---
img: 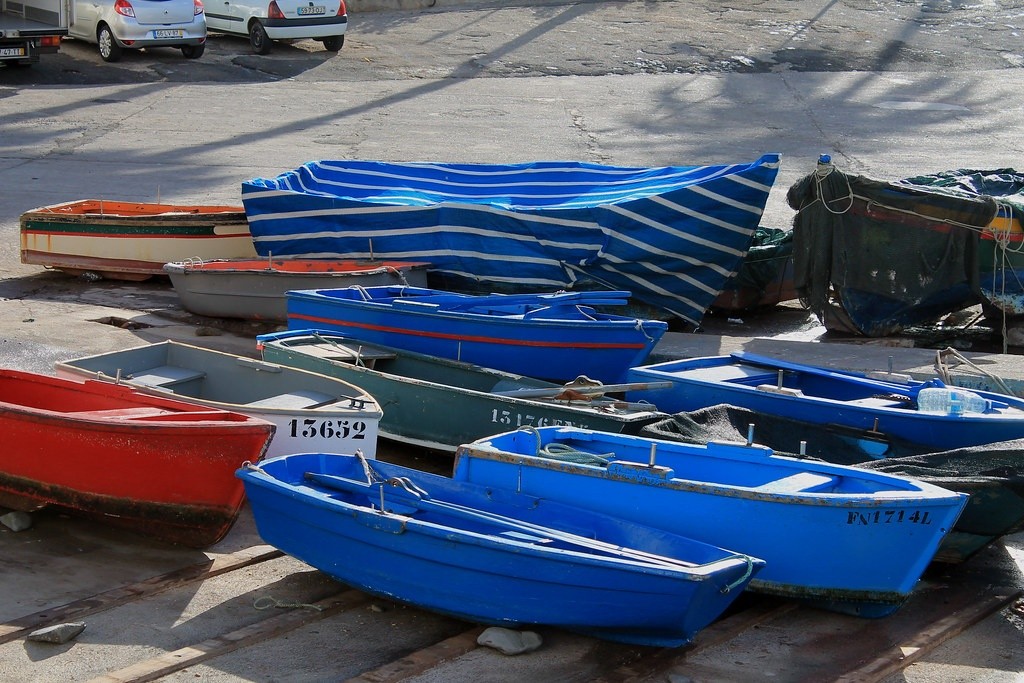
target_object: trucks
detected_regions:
[0,0,68,70]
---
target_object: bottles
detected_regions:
[917,388,991,415]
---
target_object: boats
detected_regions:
[234,449,768,653]
[241,151,782,326]
[0,369,278,550]
[623,351,1024,459]
[19,197,260,286]
[640,401,1024,567]
[786,153,1023,340]
[256,328,670,454]
[52,339,384,460]
[285,284,669,400]
[163,257,431,325]
[451,423,971,619]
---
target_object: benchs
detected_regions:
[123,365,205,398]
[847,397,907,408]
[755,472,842,493]
[676,364,804,394]
[291,342,397,370]
[246,390,336,409]
[69,407,180,420]
[496,530,552,546]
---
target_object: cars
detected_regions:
[201,0,348,56]
[63,0,208,62]
